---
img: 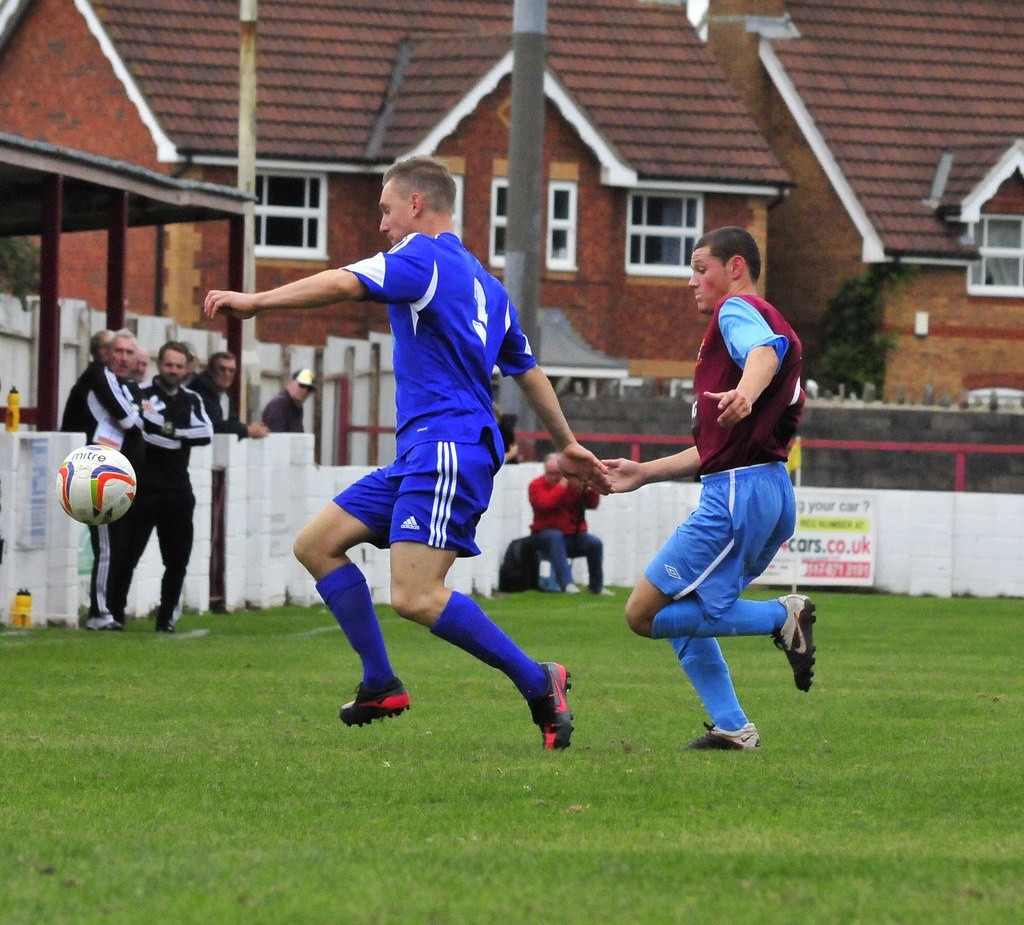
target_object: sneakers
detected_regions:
[769,594,816,692]
[339,677,410,727]
[526,662,574,749]
[682,722,759,750]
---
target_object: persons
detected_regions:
[602,227,817,750]
[201,156,612,750]
[57,328,316,633]
[528,453,616,595]
[492,401,520,463]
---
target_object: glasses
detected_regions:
[298,383,315,393]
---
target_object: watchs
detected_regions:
[163,421,174,435]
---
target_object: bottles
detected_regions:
[13,588,32,629]
[5,385,20,432]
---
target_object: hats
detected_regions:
[293,367,316,387]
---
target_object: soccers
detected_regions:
[55,444,138,526]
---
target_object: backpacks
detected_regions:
[500,538,538,591]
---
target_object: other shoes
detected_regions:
[87,621,124,631]
[566,582,581,594]
[587,586,614,594]
[156,624,176,632]
